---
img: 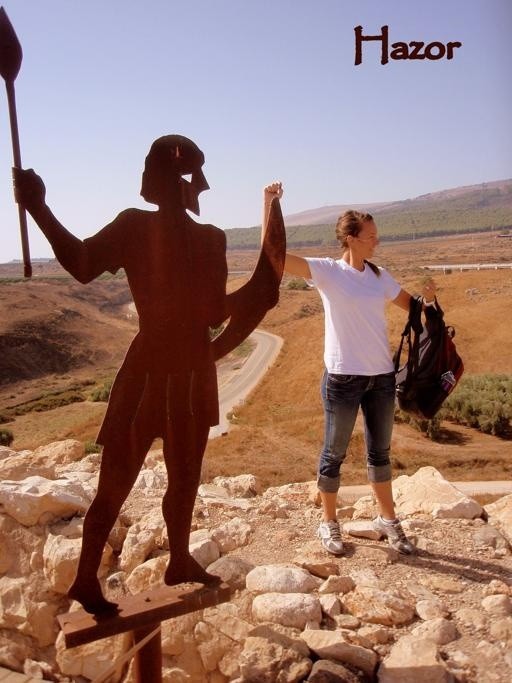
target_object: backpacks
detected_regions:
[391,294,466,421]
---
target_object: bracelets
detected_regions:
[422,297,435,306]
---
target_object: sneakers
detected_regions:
[315,520,347,554]
[371,513,415,555]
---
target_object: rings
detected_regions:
[426,285,430,290]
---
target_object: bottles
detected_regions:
[440,370,457,395]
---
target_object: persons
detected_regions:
[11,131,283,620]
[260,177,439,556]
